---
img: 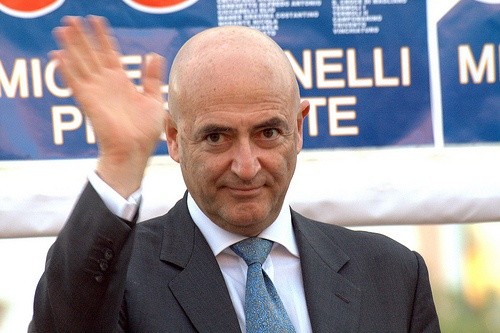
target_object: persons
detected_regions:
[27,11,442,333]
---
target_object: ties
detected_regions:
[230,237,296,333]
